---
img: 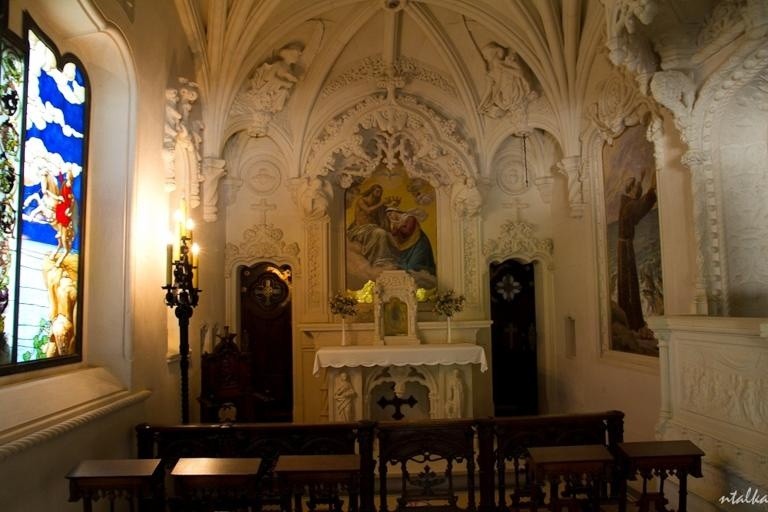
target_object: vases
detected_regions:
[342,318,345,346]
[447,316,451,343]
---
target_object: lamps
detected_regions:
[162,211,203,425]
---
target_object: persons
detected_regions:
[354,184,435,276]
[333,372,356,423]
[617,169,656,330]
[48,167,79,239]
[477,40,521,115]
[264,46,303,88]
[446,368,466,419]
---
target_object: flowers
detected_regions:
[428,290,466,317]
[328,290,360,318]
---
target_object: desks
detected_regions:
[313,344,488,422]
[616,440,705,512]
[65,454,361,512]
[526,445,614,512]
